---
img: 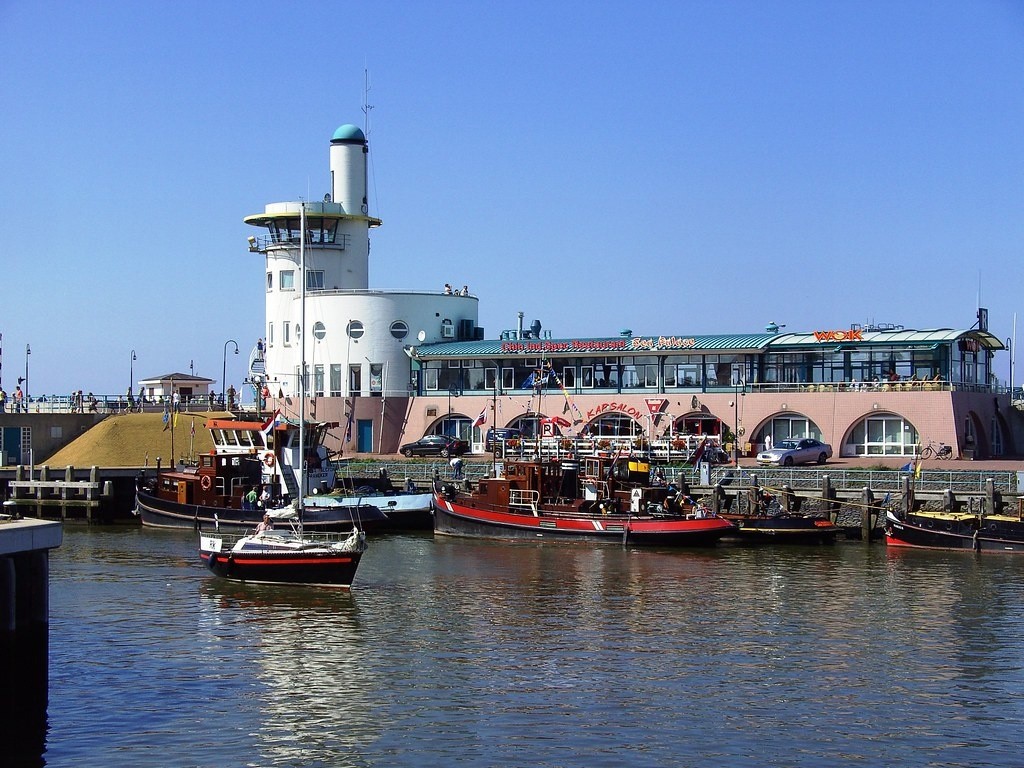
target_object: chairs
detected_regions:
[800,374,940,392]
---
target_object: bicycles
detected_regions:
[921,436,953,460]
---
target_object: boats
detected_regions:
[182,411,437,526]
[134,449,390,537]
[886,504,1024,552]
[578,454,845,541]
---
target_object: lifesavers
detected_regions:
[587,478,596,486]
[470,490,481,499]
[264,452,275,466]
[201,475,212,489]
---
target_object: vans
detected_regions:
[486,426,533,458]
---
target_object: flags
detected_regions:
[472,405,487,428]
[882,490,890,508]
[900,461,912,472]
[521,370,549,389]
[915,461,922,479]
[685,440,706,465]
[261,409,281,436]
[163,409,178,431]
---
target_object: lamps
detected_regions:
[388,501,397,506]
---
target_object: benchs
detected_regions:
[107,403,137,413]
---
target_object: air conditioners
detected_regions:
[442,325,454,337]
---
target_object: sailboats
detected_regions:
[432,345,738,547]
[197,200,368,593]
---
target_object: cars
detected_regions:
[755,438,835,465]
[399,434,470,457]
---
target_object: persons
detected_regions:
[653,435,662,450]
[257,337,266,362]
[0,385,48,414]
[71,390,86,414]
[890,368,899,386]
[89,392,99,413]
[637,430,647,440]
[109,387,172,415]
[450,458,463,480]
[241,486,271,511]
[190,385,236,404]
[255,375,270,410]
[444,283,469,297]
[849,379,880,392]
[173,389,181,414]
[764,433,771,450]
[255,514,274,535]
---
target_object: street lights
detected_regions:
[448,380,460,461]
[734,377,746,467]
[26,343,32,413]
[222,340,240,409]
[130,350,135,398]
[190,359,194,375]
